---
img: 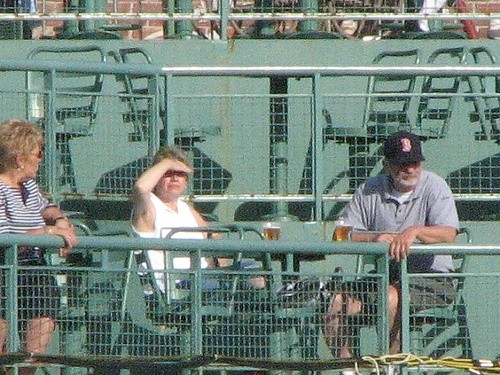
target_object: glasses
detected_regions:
[27,151,42,158]
[164,171,185,176]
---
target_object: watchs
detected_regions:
[53,216,70,226]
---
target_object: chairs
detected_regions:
[108,46,219,152]
[65,231,134,375]
[24,45,106,195]
[207,225,319,374]
[322,227,472,361]
[368,46,466,192]
[461,46,500,145]
[162,226,244,373]
[321,50,420,195]
[111,230,180,374]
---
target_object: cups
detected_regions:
[334,221,354,242]
[263,223,281,241]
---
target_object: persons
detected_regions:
[126,143,322,312]
[0,117,77,375]
[315,129,461,375]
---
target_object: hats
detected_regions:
[384,132,425,166]
